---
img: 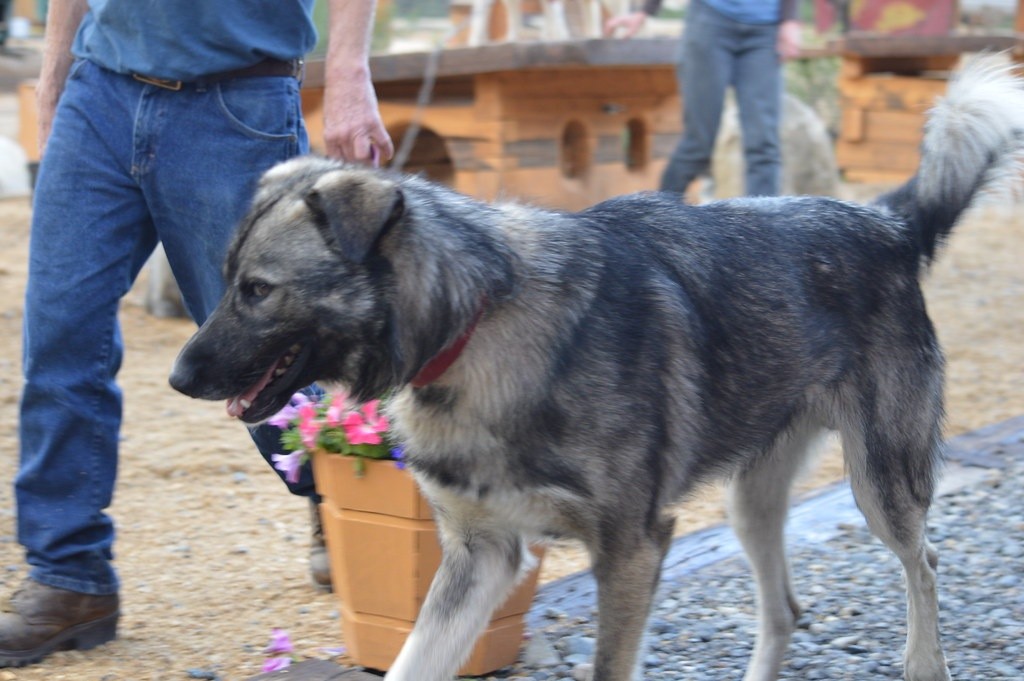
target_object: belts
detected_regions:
[128,62,294,77]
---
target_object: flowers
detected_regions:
[262,380,410,487]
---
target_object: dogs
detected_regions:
[165,47,1021,681]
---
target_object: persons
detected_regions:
[0,0,396,668]
[600,0,805,200]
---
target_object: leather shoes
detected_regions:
[0,577,119,668]
[306,495,335,591]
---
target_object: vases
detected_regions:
[308,445,557,679]
